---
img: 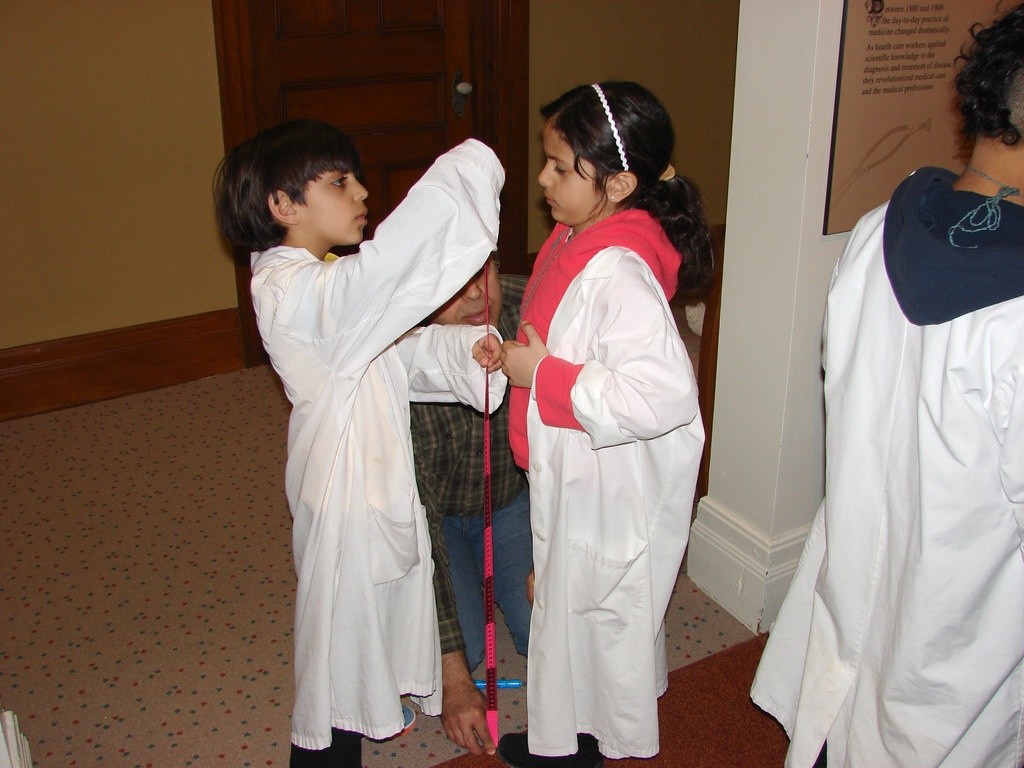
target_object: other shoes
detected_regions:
[498,729,604,768]
[371,703,417,739]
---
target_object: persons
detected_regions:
[748,1,1024,768]
[215,82,714,767]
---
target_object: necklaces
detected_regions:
[518,215,573,325]
[949,162,1024,248]
